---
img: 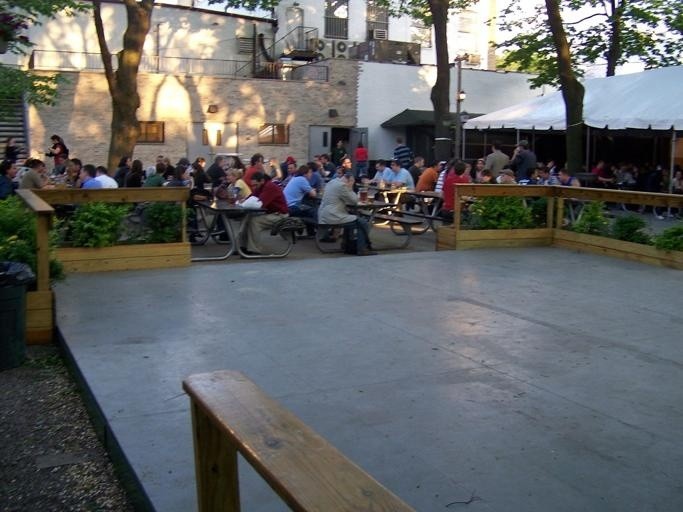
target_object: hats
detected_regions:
[176,158,189,166]
[287,157,295,163]
[515,140,528,147]
[499,169,513,177]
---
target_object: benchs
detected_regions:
[280,199,444,255]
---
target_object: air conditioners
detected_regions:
[309,38,353,62]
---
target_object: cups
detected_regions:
[321,183,326,195]
[358,187,369,204]
[228,189,237,204]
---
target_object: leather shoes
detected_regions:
[357,250,378,255]
[233,247,247,256]
[240,251,259,259]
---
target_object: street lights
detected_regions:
[454,53,469,163]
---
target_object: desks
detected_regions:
[197,201,265,262]
[355,183,444,199]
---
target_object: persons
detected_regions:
[352,142,367,174]
[408,156,583,225]
[592,161,681,219]
[392,137,415,167]
[43,134,68,166]
[5,136,21,163]
[330,138,347,163]
[483,140,509,177]
[508,139,535,177]
[1,154,118,216]
[114,155,414,253]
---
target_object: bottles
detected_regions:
[377,179,385,188]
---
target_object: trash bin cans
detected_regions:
[0,261,36,370]
